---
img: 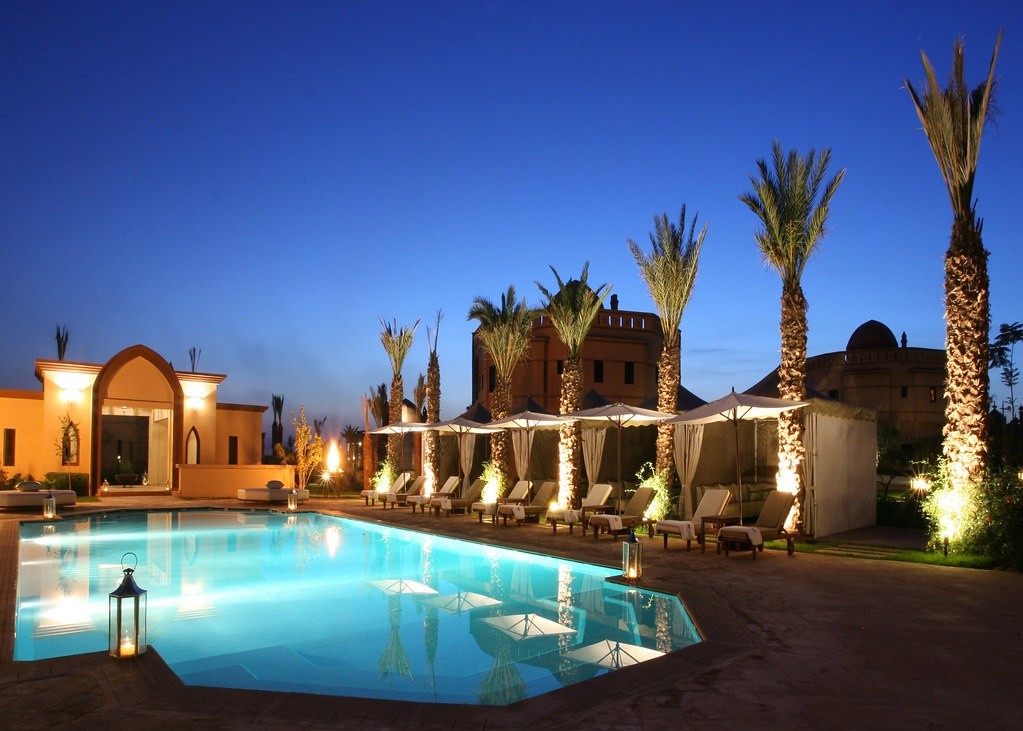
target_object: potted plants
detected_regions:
[114,460,139,483]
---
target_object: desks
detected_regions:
[429,493,452,518]
[582,505,616,540]
[701,515,741,555]
[496,497,527,528]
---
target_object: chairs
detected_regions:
[360,472,560,528]
[716,490,799,561]
[588,487,659,542]
[546,483,614,536]
[656,490,733,552]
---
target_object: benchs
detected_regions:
[597,481,632,515]
[0,489,77,511]
[696,485,767,527]
[237,488,309,503]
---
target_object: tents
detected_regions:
[438,382,878,538]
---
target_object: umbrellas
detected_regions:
[477,561,577,641]
[557,399,678,514]
[421,417,505,498]
[366,543,440,596]
[661,386,813,525]
[418,570,503,616]
[363,421,427,493]
[476,411,580,505]
[558,605,667,671]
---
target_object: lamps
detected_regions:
[622,519,644,585]
[44,487,56,520]
[622,587,642,633]
[107,552,149,665]
[103,478,109,492]
[288,488,298,511]
[165,480,171,491]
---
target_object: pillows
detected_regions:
[266,480,284,489]
[15,481,41,492]
[532,479,548,495]
[702,483,777,504]
[607,479,641,499]
